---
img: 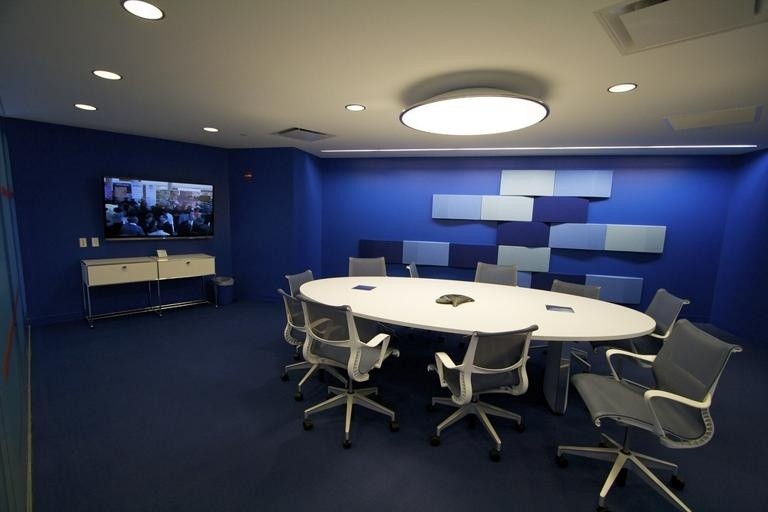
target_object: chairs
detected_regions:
[529,280,602,369]
[295,295,400,449]
[474,262,517,286]
[406,262,419,278]
[349,257,386,276]
[558,319,743,512]
[277,289,349,402]
[428,325,538,462]
[563,289,690,413]
[285,270,313,297]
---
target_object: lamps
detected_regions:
[399,87,550,135]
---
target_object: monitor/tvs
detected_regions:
[103,175,216,241]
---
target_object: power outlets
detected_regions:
[80,238,87,247]
[92,238,99,247]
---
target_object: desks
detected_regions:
[300,277,656,414]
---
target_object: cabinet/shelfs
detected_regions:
[80,253,218,328]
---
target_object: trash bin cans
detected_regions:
[212,276,235,308]
